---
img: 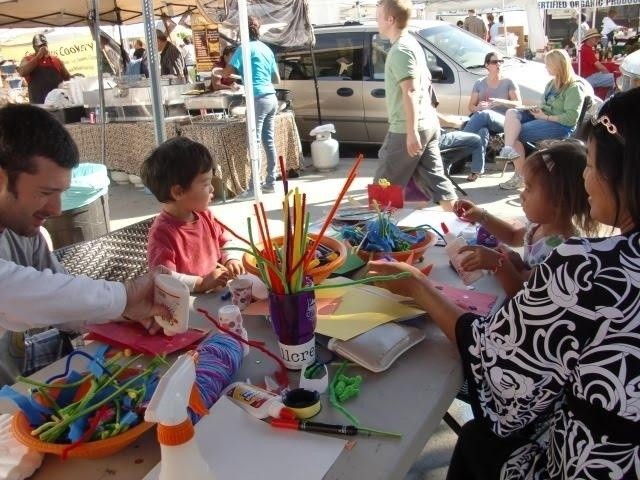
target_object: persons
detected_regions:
[452,137,602,298]
[371,1,459,209]
[439,7,629,182]
[207,44,238,94]
[139,135,247,294]
[128,29,197,86]
[220,15,282,203]
[19,33,75,104]
[0,102,178,393]
[364,84,640,480]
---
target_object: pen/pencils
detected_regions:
[257,416,357,435]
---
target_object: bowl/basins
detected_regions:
[241,234,347,291]
[10,365,164,460]
[343,225,438,265]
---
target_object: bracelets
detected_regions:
[494,251,508,274]
[479,207,488,230]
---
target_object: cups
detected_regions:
[226,279,254,310]
[267,275,316,371]
[218,304,251,359]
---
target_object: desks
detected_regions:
[0,243,507,480]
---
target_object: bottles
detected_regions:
[441,222,483,285]
[457,226,478,246]
[222,382,297,422]
[150,274,191,340]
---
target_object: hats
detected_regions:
[33,34,48,46]
[581,29,601,43]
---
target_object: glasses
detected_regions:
[490,59,505,65]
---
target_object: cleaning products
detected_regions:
[144,349,214,480]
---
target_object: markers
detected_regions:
[305,236,334,254]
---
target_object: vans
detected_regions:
[258,19,595,163]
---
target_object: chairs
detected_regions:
[48,212,155,290]
[572,91,604,147]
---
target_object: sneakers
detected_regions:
[261,184,275,192]
[234,190,262,201]
[499,172,525,190]
[494,147,521,161]
